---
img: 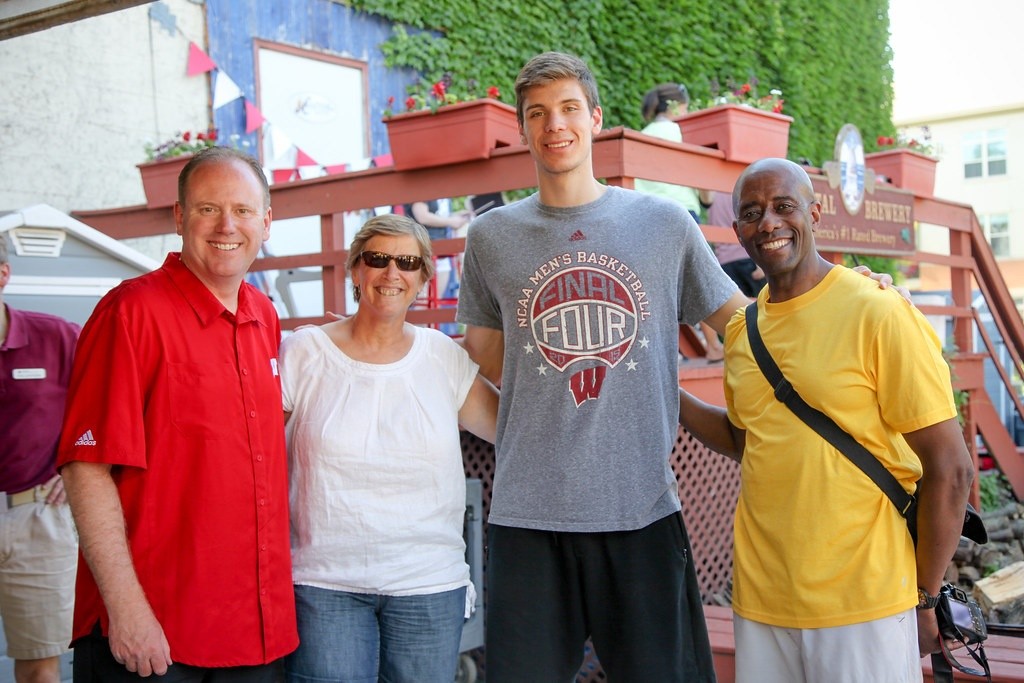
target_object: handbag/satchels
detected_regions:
[904,479,988,552]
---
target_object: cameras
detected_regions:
[934,582,987,645]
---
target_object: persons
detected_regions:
[679,159,973,683]
[403,198,466,335]
[0,234,82,683]
[634,83,768,365]
[293,47,914,683]
[54,143,301,683]
[277,213,501,683]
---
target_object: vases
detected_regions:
[677,104,795,165]
[134,155,194,210]
[863,149,939,199]
[382,98,524,171]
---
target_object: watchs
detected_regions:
[915,587,941,610]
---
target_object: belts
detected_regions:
[7,485,54,509]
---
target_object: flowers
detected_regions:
[146,129,217,161]
[870,135,927,153]
[703,75,782,113]
[383,74,502,115]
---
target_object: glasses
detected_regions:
[359,251,425,271]
